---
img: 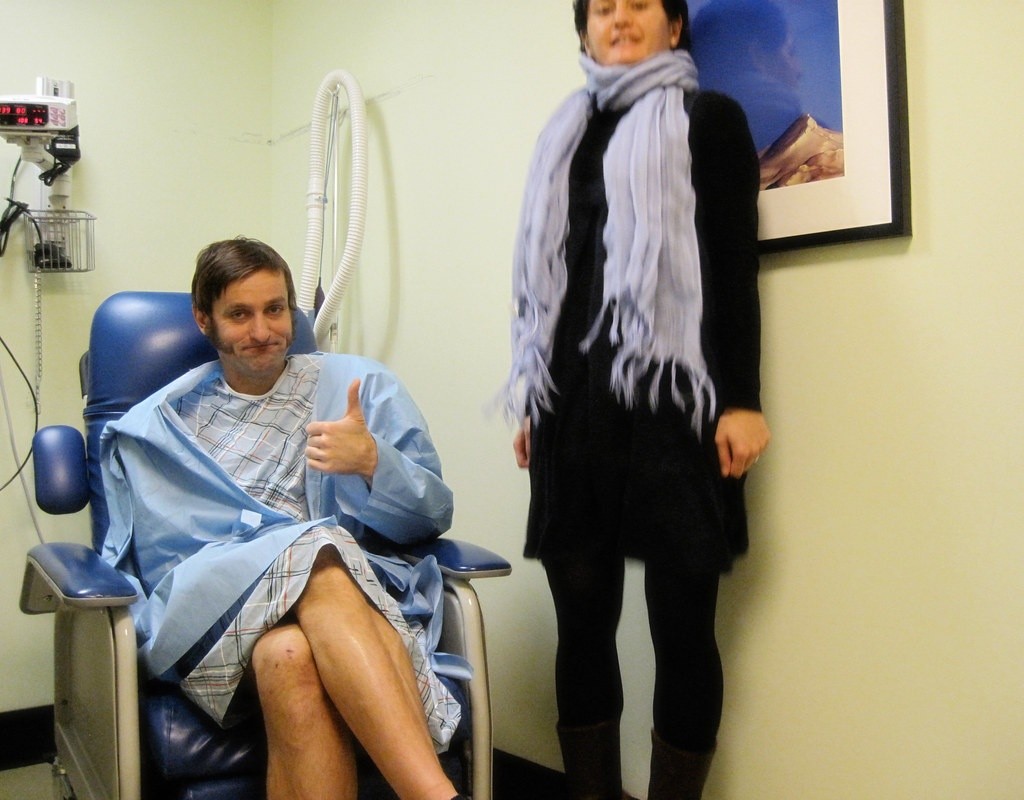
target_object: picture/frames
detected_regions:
[674,0,913,255]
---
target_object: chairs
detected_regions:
[18,291,513,800]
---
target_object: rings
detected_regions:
[755,457,759,463]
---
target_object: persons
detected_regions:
[99,239,462,800]
[512,0,768,800]
